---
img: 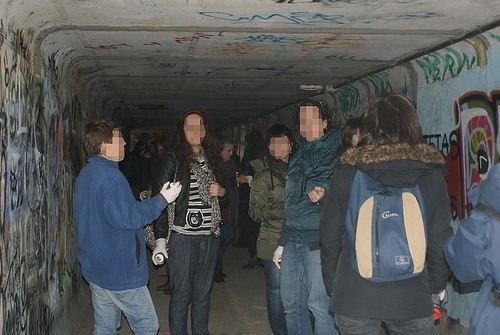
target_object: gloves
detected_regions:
[159,181,182,204]
[273,245,284,269]
[152,239,168,265]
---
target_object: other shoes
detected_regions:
[243,258,262,270]
[232,242,247,248]
[433,303,441,325]
[215,272,229,283]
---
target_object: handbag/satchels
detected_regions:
[139,152,178,251]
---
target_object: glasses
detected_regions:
[224,148,235,152]
[303,100,326,116]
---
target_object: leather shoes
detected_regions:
[156,282,170,294]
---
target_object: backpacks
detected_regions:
[345,170,428,283]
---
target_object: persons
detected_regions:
[238,129,268,269]
[248,123,296,334]
[341,116,367,150]
[272,100,344,335]
[320,92,453,335]
[215,134,245,283]
[73,120,184,335]
[442,163,500,335]
[151,110,233,335]
